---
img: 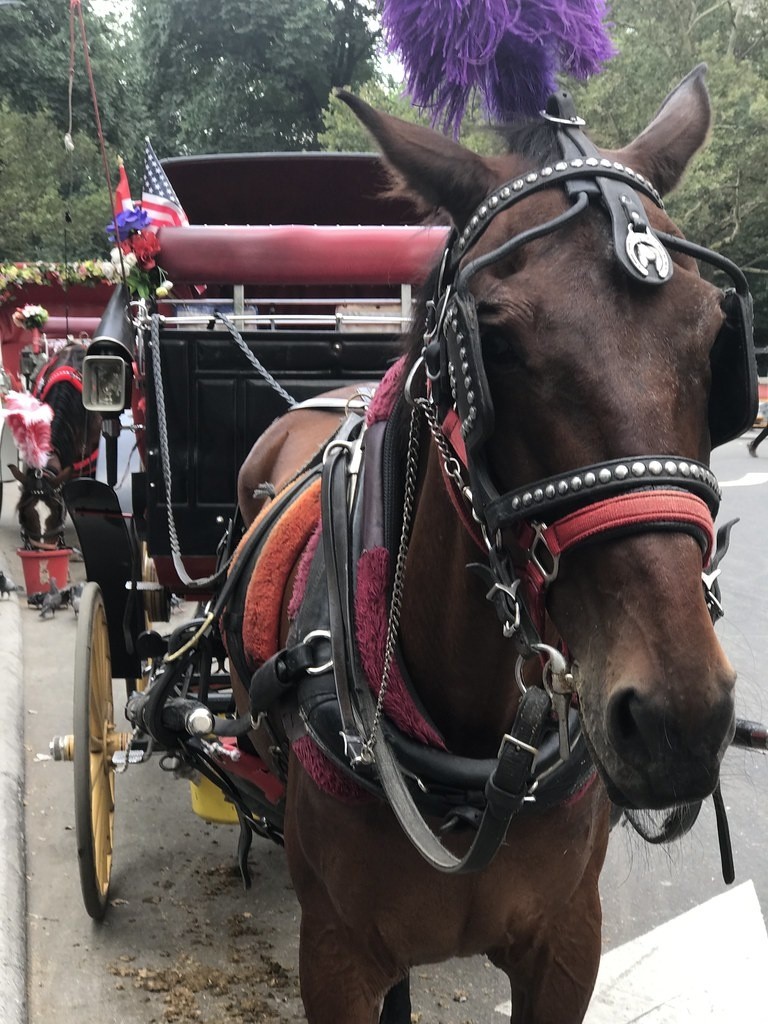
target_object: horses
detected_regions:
[7,341,103,554]
[208,58,741,1024]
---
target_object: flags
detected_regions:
[114,165,135,219]
[143,137,209,294]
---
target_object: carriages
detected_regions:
[1,0,768,1024]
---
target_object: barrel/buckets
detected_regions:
[17,546,71,599]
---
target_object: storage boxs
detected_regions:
[15,547,73,597]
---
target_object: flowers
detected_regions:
[0,207,174,300]
[12,303,48,333]
